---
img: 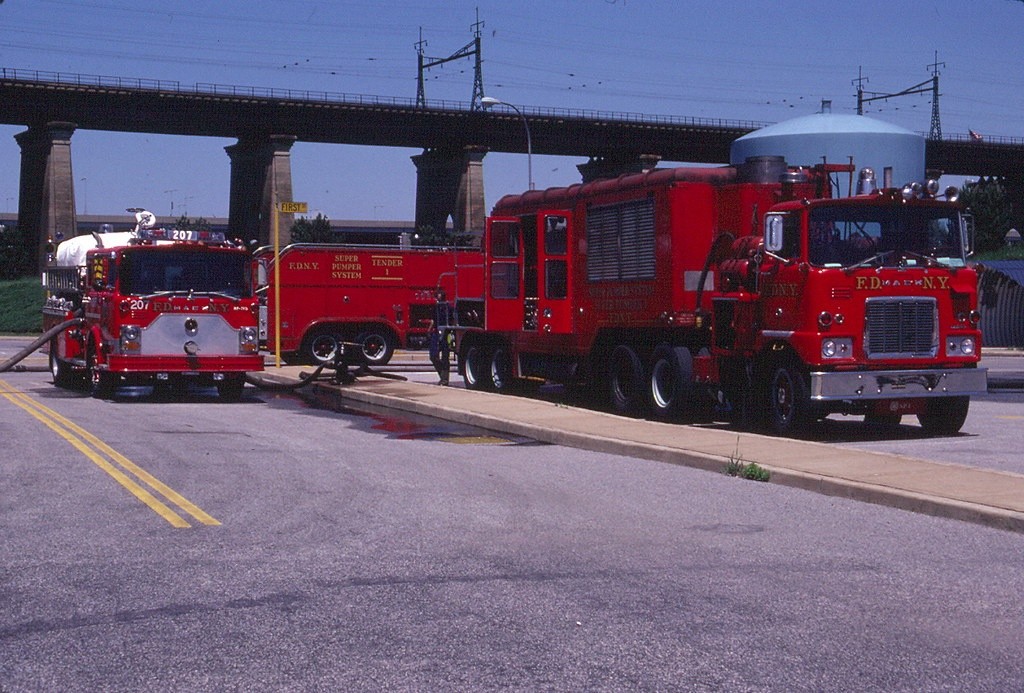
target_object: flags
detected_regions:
[969,129,983,141]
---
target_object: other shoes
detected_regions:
[439,378,448,386]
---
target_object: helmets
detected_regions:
[434,291,445,301]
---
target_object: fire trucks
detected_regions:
[40,207,268,402]
[249,245,486,366]
[436,154,990,439]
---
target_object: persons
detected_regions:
[429,289,454,386]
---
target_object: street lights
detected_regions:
[480,97,532,191]
